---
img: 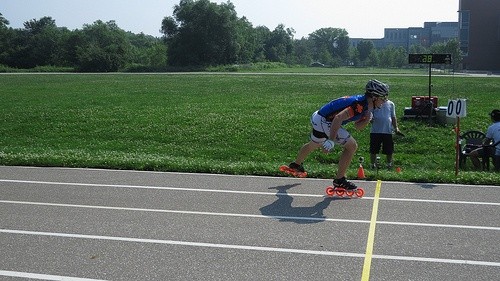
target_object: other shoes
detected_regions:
[371,164,377,169]
[387,164,393,171]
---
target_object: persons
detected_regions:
[470,109,500,171]
[279,79,390,197]
[369,98,400,170]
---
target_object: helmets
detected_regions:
[366,79,389,96]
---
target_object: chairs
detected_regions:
[482,140,500,173]
[459,130,490,171]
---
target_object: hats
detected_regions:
[488,109,500,115]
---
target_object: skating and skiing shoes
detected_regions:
[326,176,364,198]
[279,162,308,177]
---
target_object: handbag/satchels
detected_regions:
[483,145,496,157]
